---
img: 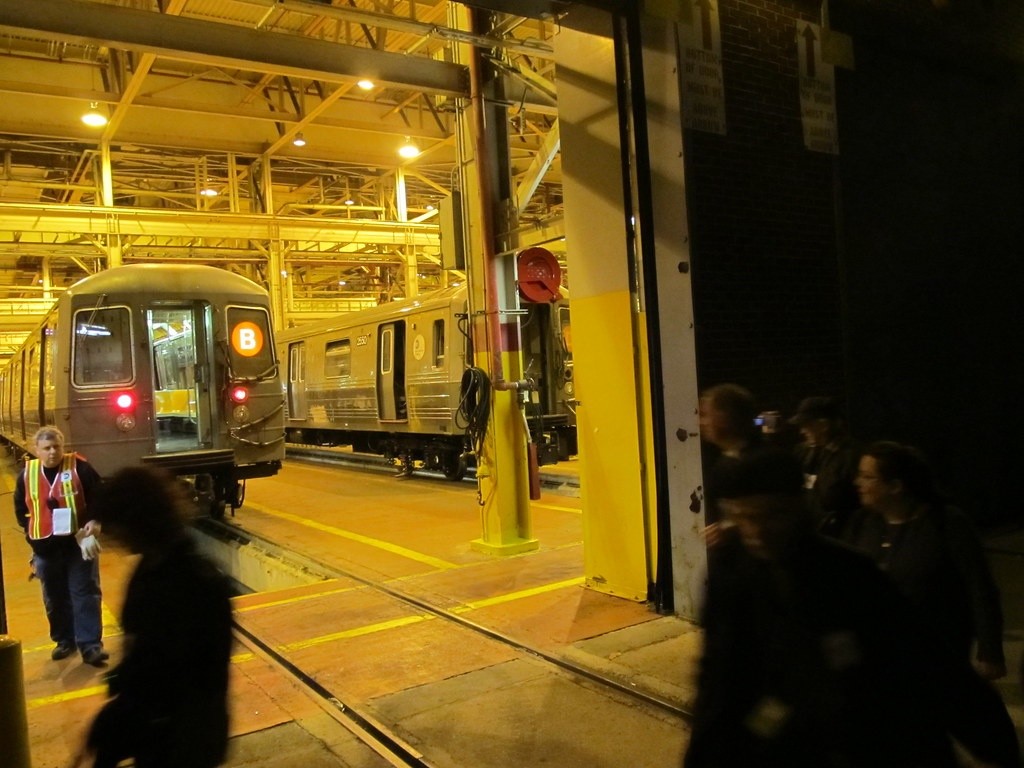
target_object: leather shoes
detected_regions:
[81,648,110,665]
[51,641,78,661]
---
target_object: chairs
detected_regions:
[154,389,196,419]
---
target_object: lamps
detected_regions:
[294,131,305,146]
[82,58,107,128]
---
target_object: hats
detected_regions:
[785,397,832,427]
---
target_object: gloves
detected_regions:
[73,528,103,561]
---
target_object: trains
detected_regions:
[274,271,577,484]
[1,265,287,525]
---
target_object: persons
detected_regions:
[684,384,1024,768]
[12,425,112,664]
[82,467,233,768]
